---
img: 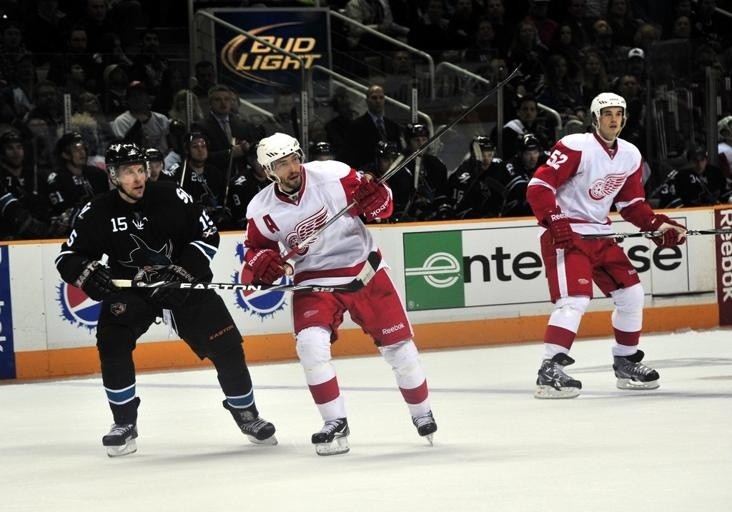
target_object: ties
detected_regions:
[221,117,233,144]
[376,120,386,139]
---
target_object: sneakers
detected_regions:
[613,350,660,384]
[311,417,352,444]
[410,410,438,437]
[537,352,584,390]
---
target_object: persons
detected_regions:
[0,1,732,244]
[54,144,280,448]
[524,90,689,394]
[240,132,439,448]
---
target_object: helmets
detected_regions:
[105,141,151,187]
[144,146,167,163]
[0,130,28,167]
[375,138,400,160]
[716,115,732,142]
[255,132,306,185]
[56,129,89,165]
[177,126,209,153]
[310,142,337,160]
[470,90,640,166]
[404,123,428,144]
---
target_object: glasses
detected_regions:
[666,153,682,159]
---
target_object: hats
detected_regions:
[103,61,122,86]
[127,80,146,95]
[627,47,645,61]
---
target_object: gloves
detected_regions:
[66,254,129,306]
[543,205,575,249]
[132,264,201,306]
[641,214,688,249]
[351,175,390,222]
[246,247,288,287]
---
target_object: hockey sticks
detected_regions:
[111,251,381,293]
[281,61,523,263]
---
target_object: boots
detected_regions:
[220,398,277,440]
[101,397,140,446]
